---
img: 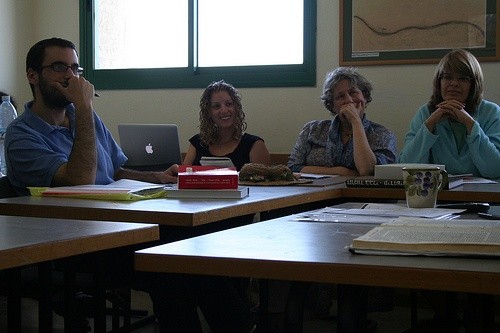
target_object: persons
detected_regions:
[398,49,500,180]
[287,69,401,178]
[4,37,260,333]
[182,79,272,171]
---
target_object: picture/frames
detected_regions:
[338,0,500,66]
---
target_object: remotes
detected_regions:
[434,202,490,212]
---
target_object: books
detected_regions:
[344,216,500,258]
[345,176,464,190]
[164,165,249,199]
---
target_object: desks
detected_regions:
[0,169,500,333]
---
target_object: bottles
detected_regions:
[0,96,18,176]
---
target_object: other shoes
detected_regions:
[53,305,92,332]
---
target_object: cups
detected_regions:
[401,167,448,208]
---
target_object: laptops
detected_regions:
[117,123,183,166]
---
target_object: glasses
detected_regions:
[35,62,84,76]
[437,71,474,85]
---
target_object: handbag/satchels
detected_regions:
[239,162,293,182]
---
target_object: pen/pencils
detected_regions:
[64,80,100,97]
